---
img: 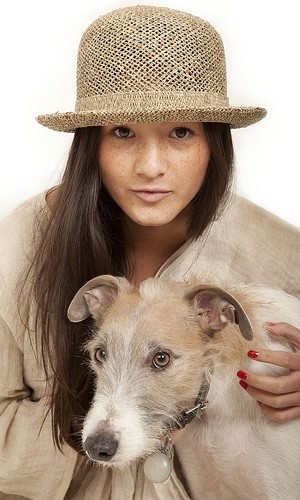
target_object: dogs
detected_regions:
[67,275,300,499]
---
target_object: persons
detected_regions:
[0,6,299,500]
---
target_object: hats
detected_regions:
[35,6,267,133]
